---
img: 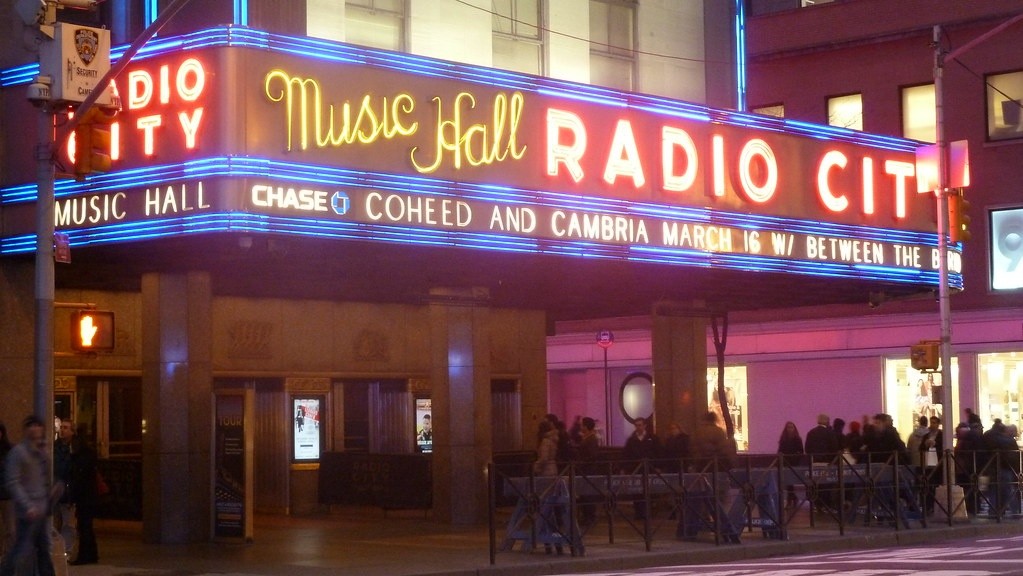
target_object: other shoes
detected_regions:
[70,555,99,565]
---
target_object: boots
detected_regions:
[793,495,799,510]
[784,494,792,509]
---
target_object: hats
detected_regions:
[818,414,830,424]
[850,421,860,431]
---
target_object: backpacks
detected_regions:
[556,430,572,456]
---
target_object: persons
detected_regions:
[417,414,432,440]
[55,416,61,440]
[530,414,599,555]
[907,407,1020,519]
[776,421,804,511]
[806,414,906,524]
[688,413,726,472]
[0,420,100,576]
[620,417,689,519]
